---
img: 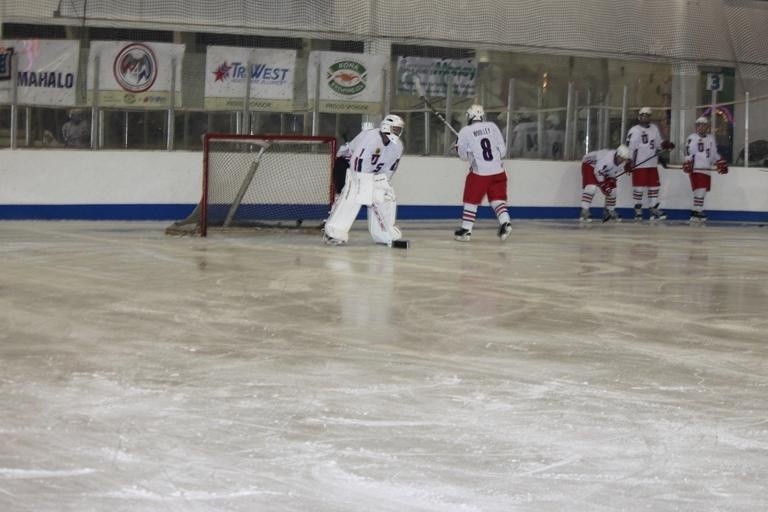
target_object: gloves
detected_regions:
[623,159,639,173]
[683,161,692,173]
[660,140,676,151]
[716,155,728,174]
[598,177,617,197]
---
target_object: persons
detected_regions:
[682,116,728,223]
[511,109,538,159]
[578,145,631,223]
[607,110,622,149]
[62,108,91,148]
[624,106,676,220]
[320,114,408,251]
[571,109,593,161]
[542,114,564,160]
[453,103,513,241]
[495,110,508,144]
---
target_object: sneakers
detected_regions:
[454,229,473,237]
[687,211,709,219]
[497,221,512,237]
[648,202,667,218]
[605,208,621,220]
[632,203,644,217]
[580,209,593,219]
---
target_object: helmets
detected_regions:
[695,116,708,125]
[380,115,406,146]
[616,144,631,160]
[637,107,653,122]
[465,104,487,123]
